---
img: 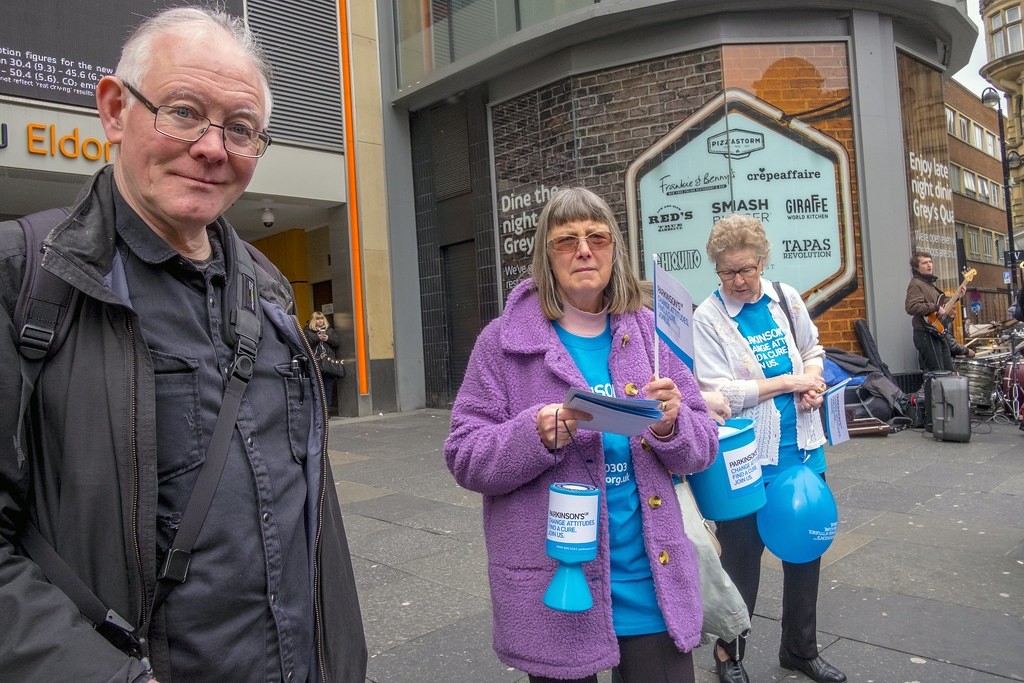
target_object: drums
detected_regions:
[952,358,1024,420]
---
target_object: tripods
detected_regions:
[993,329,1024,424]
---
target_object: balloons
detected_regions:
[757,462,840,565]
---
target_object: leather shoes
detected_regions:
[778,643,847,683]
[713,642,749,683]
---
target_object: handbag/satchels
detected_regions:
[320,356,344,378]
[669,467,751,644]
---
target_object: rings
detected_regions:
[661,402,667,411]
[817,388,822,392]
[822,383,824,388]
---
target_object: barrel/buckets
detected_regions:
[687,414,769,520]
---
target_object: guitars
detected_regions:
[922,265,978,339]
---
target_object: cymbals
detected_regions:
[968,319,1024,360]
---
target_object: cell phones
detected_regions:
[320,330,326,335]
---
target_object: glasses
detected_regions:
[546,231,613,253]
[121,79,272,158]
[716,257,761,281]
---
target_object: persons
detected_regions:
[302,311,340,419]
[0,3,369,683]
[701,390,732,426]
[692,212,849,683]
[443,185,720,683]
[906,252,976,435]
